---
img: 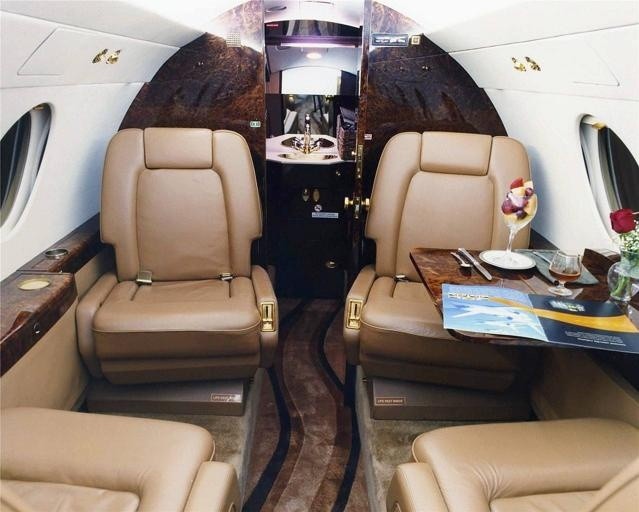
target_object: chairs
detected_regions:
[343,131,530,420]
[74,127,280,418]
[1,408,242,512]
[387,416,639,512]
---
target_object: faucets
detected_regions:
[305,112,310,123]
[303,124,310,154]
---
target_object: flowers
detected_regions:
[609,209,638,250]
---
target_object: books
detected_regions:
[440,282,639,354]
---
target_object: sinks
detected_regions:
[278,152,337,161]
[282,136,335,147]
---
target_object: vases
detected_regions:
[608,251,639,302]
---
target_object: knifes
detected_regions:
[458,247,493,281]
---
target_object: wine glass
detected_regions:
[547,249,582,297]
[493,192,538,266]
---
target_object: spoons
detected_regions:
[450,252,473,268]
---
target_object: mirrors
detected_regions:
[268,64,359,150]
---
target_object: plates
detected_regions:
[479,249,537,271]
